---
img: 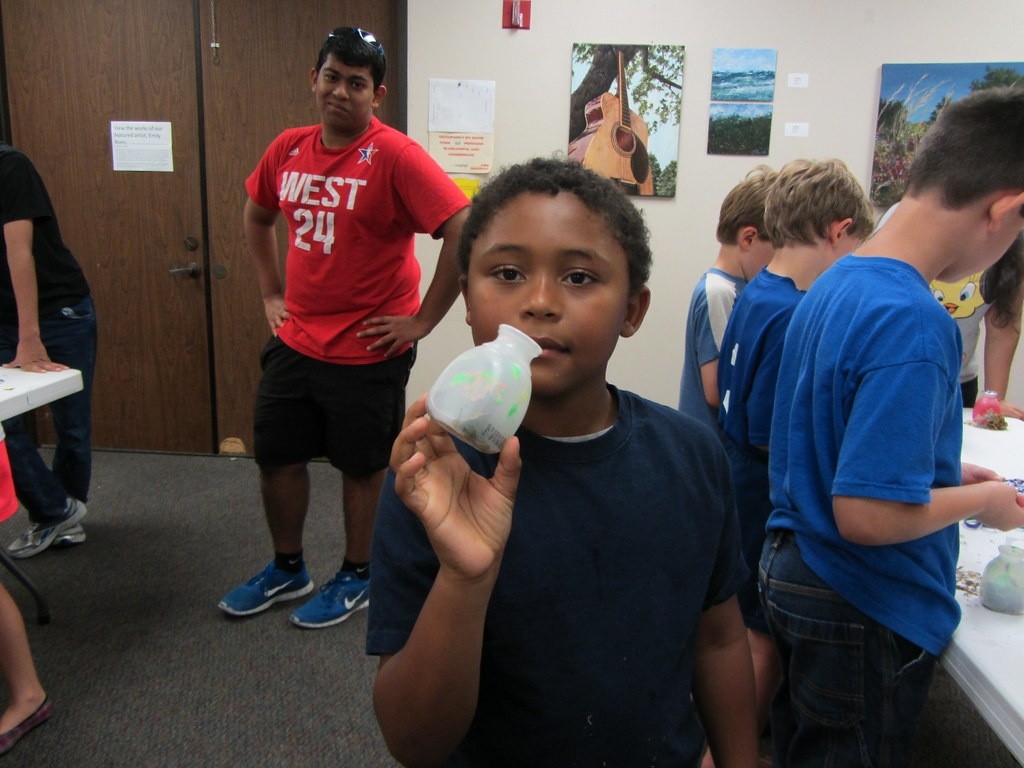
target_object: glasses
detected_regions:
[326,25,384,56]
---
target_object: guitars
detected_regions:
[569,47,655,195]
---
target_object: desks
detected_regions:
[0,364,82,624]
[934,408,1024,768]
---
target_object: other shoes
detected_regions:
[0,694,54,757]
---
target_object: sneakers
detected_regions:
[288,568,371,629]
[6,491,89,558]
[218,558,315,617]
[50,522,87,548]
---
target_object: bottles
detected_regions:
[979,544,1024,613]
[971,390,1002,426]
[426,323,542,455]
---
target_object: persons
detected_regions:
[0,146,98,559]
[217,27,471,628]
[701,158,876,768]
[0,424,55,755]
[678,163,778,431]
[365,156,762,768]
[877,201,1024,422]
[755,84,1024,768]
